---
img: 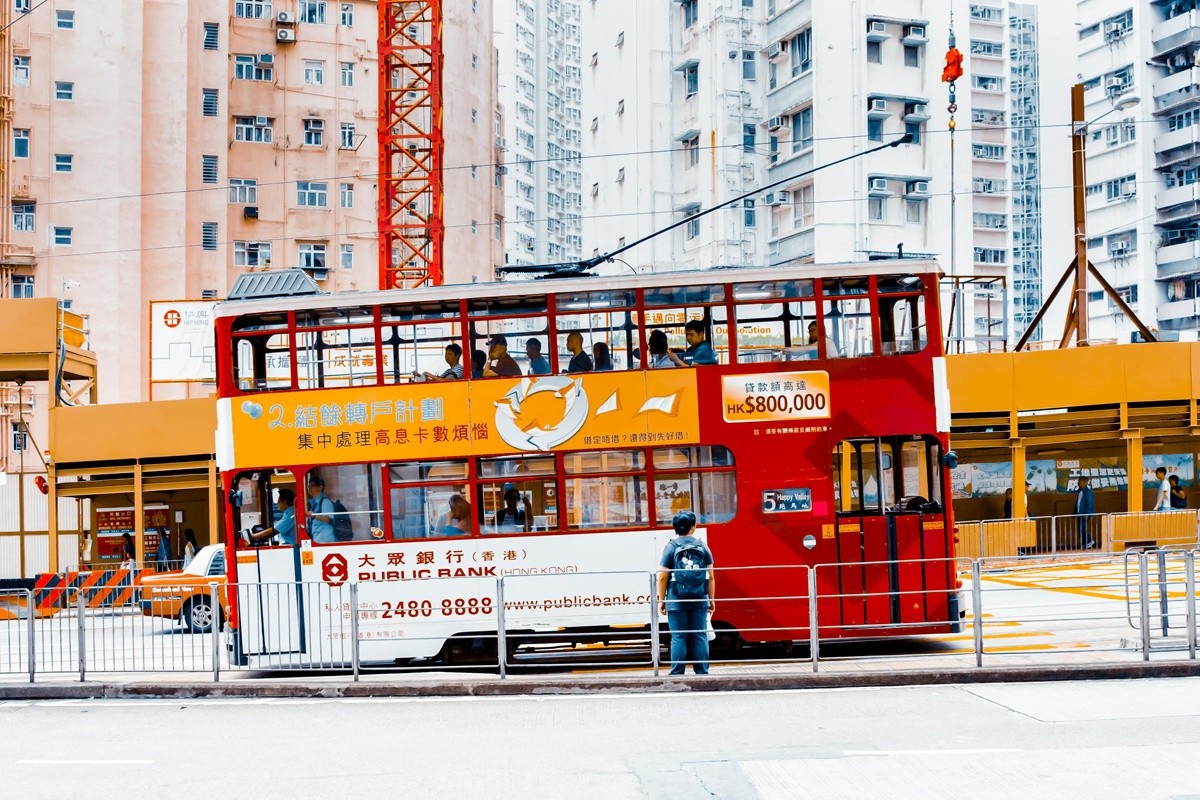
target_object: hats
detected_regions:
[484,336,507,347]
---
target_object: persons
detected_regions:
[525,338,550,374]
[307,477,338,542]
[483,335,522,378]
[647,329,676,369]
[492,488,534,533]
[248,489,296,544]
[779,323,840,359]
[121,527,199,573]
[470,349,487,379]
[668,320,718,366]
[412,343,464,380]
[562,331,592,373]
[593,342,613,370]
[1003,468,1187,556]
[443,495,472,536]
[660,510,715,675]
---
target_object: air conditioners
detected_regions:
[276,29,295,42]
[869,21,886,32]
[1105,77,1122,89]
[870,98,886,111]
[767,41,783,60]
[870,178,887,192]
[276,10,294,24]
[1104,23,1120,34]
[908,182,929,193]
[1120,181,1136,193]
[769,116,786,129]
[1112,241,1124,251]
[911,103,928,115]
[764,191,787,205]
[907,25,924,37]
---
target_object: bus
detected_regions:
[216,253,965,665]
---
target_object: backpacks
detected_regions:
[665,539,707,602]
[332,497,353,540]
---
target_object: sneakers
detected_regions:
[1085,541,1094,550]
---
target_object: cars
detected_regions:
[139,542,227,632]
